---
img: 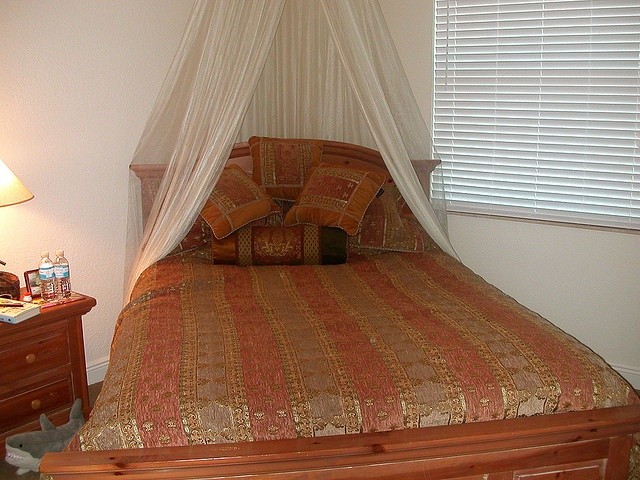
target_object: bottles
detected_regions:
[53,250,72,299]
[38,250,56,301]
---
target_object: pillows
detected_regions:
[167,218,213,257]
[350,181,425,255]
[200,162,281,241]
[248,132,324,203]
[283,159,388,236]
[208,222,350,267]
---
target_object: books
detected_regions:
[0,298,41,324]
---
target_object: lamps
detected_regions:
[0,158,36,299]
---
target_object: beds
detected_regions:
[38,137,639,476]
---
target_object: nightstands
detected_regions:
[1,286,100,462]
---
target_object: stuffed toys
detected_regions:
[4,397,87,476]
